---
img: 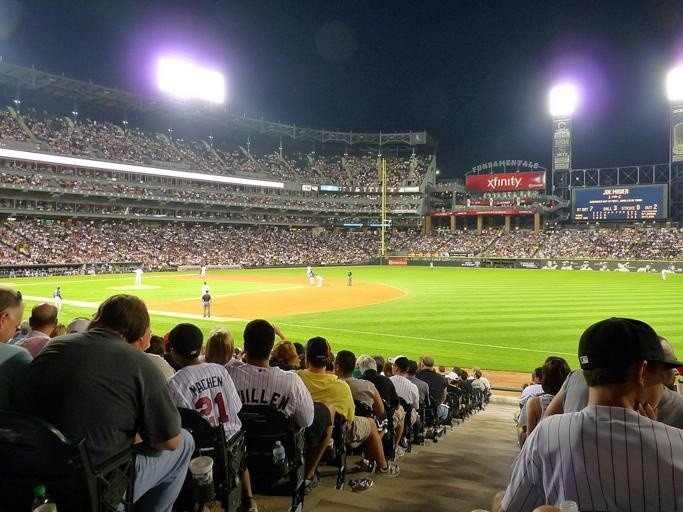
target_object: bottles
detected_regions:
[272,439,286,464]
[21,484,59,512]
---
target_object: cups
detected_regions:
[189,456,216,488]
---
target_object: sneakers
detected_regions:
[395,445,406,456]
[306,473,319,496]
[375,465,400,476]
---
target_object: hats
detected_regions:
[67,317,89,334]
[580,318,682,367]
[309,336,330,360]
[167,324,203,357]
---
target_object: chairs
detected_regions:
[0,344,491,512]
